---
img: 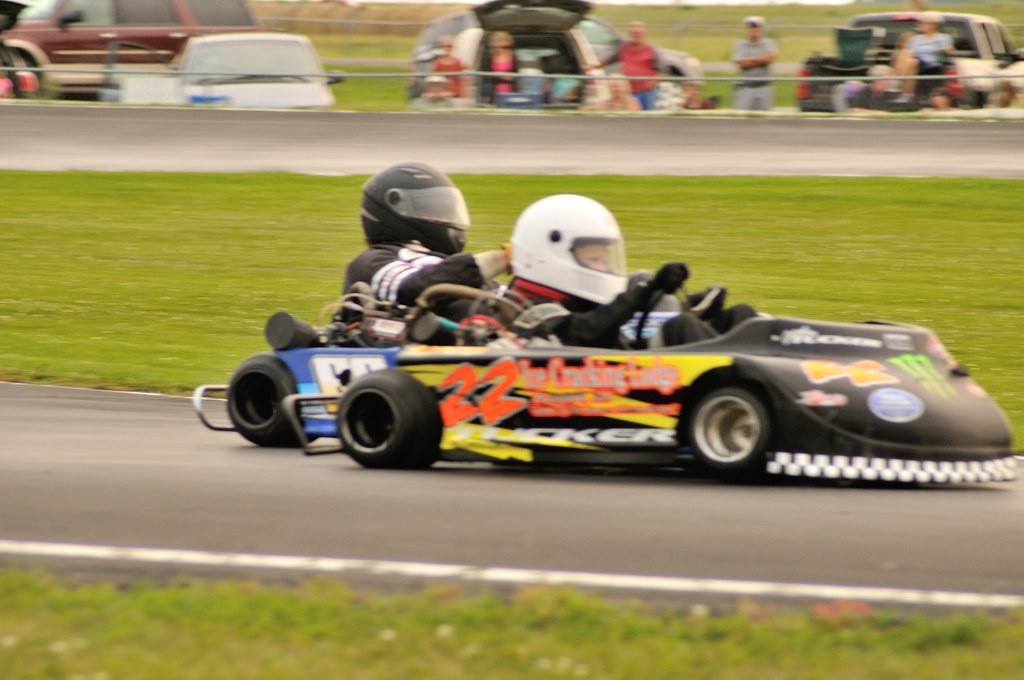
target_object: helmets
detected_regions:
[360,162,466,256]
[507,192,628,306]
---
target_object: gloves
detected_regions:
[647,262,689,292]
[695,287,727,319]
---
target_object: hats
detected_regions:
[743,14,767,28]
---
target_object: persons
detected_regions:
[848,13,1024,110]
[472,187,757,347]
[428,37,463,98]
[580,20,715,108]
[482,29,521,104]
[335,165,510,321]
[728,17,778,110]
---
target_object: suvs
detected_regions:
[1,0,264,100]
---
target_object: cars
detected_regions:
[456,0,607,112]
[405,9,710,108]
[98,31,350,110]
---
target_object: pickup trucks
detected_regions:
[792,10,1024,112]
